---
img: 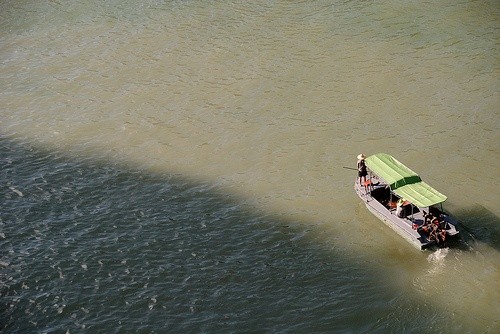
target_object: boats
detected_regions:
[353,151,460,251]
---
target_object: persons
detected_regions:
[419,209,442,244]
[357,153,368,188]
[395,198,405,219]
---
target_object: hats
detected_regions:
[357,154,366,160]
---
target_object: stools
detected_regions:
[364,181,374,192]
